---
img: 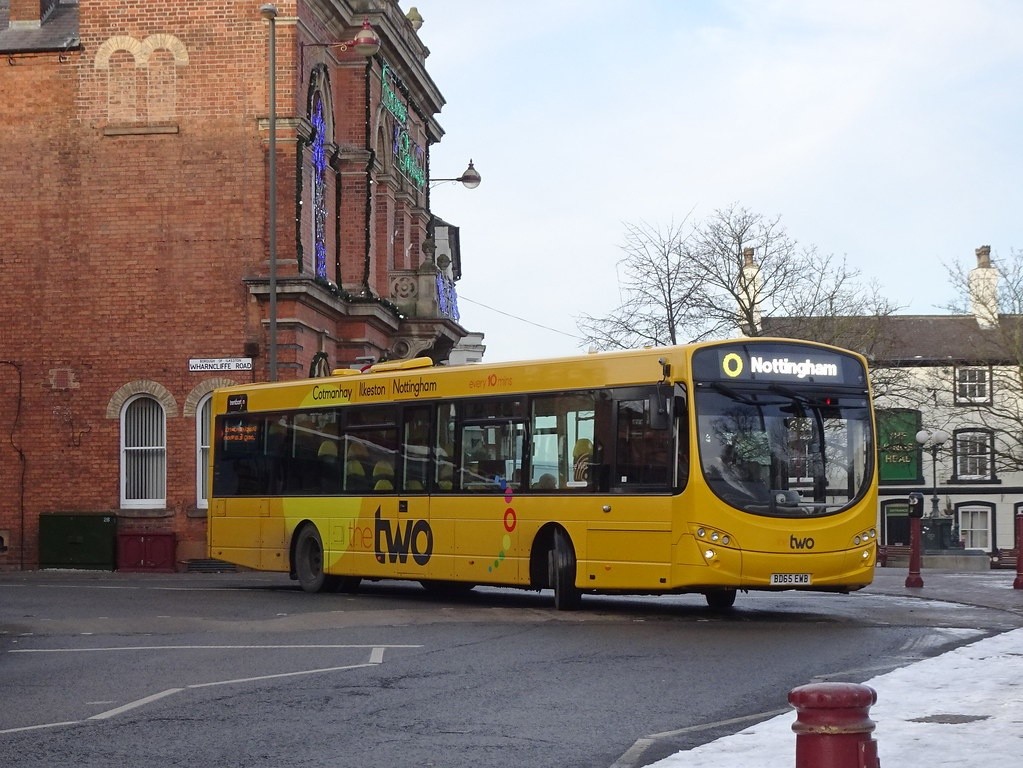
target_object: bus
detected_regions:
[207,336,882,612]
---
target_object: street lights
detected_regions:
[916,429,947,520]
[259,5,278,380]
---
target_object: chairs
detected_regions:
[221,418,800,508]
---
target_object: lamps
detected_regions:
[429,157,481,189]
[300,15,381,86]
[969,244,1001,330]
[739,248,764,336]
